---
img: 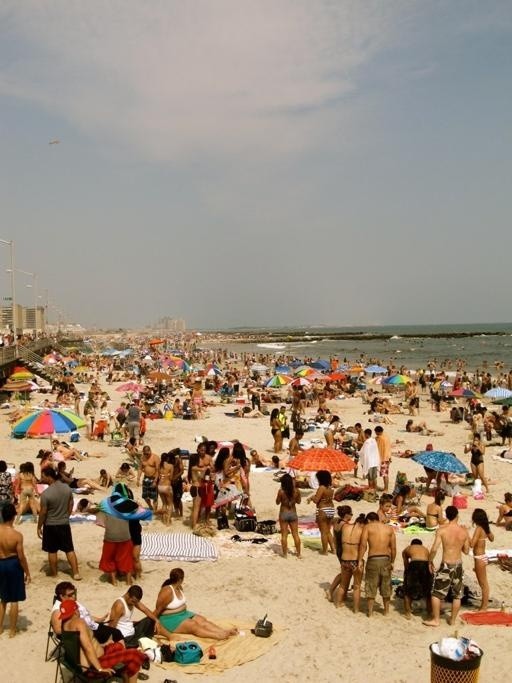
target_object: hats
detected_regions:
[59,600,78,620]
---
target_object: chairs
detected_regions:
[56,630,127,683]
[42,595,66,661]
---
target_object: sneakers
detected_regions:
[143,654,150,670]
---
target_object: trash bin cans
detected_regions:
[429,636,484,683]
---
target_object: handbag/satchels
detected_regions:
[257,520,276,534]
[234,518,257,531]
[217,515,229,530]
[192,526,216,537]
